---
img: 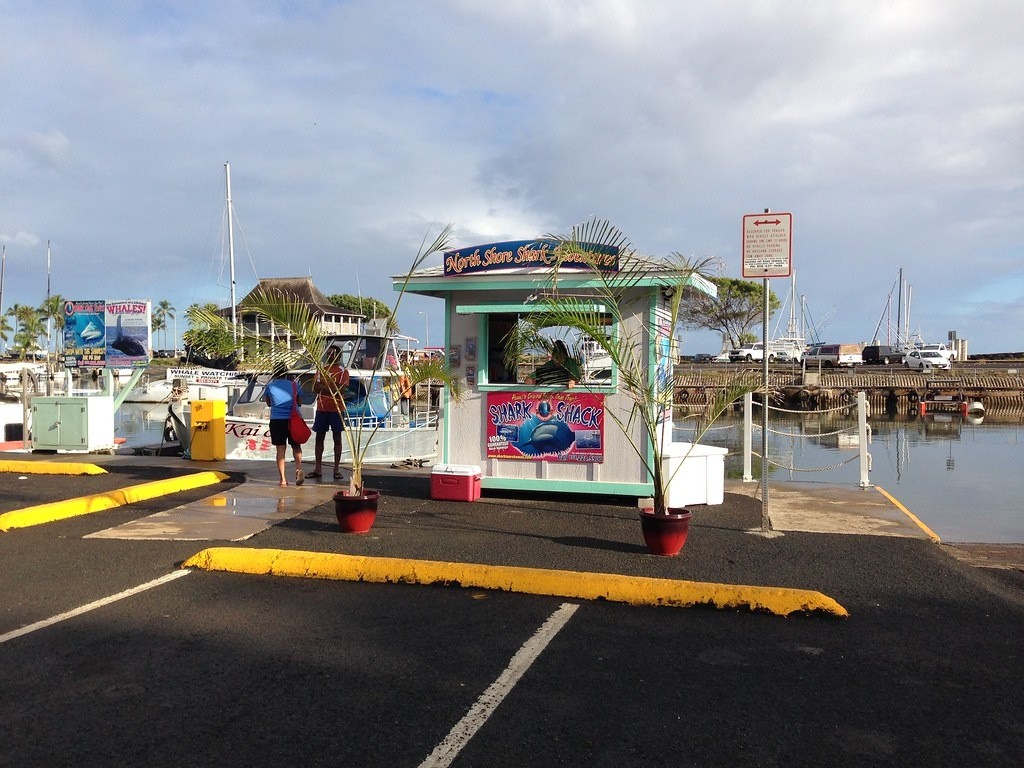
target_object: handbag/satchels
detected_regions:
[289,382,312,445]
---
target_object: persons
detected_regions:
[525,340,582,388]
[304,345,350,479]
[265,362,304,485]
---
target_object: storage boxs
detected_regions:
[430,463,482,502]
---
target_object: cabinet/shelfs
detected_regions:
[31,395,114,451]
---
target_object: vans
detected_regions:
[801,344,863,368]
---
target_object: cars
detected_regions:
[774,351,792,362]
[903,348,952,372]
[921,344,958,362]
[862,345,907,365]
[728,342,778,364]
[690,354,713,363]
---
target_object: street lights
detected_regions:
[419,311,429,347]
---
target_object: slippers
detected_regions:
[295,470,305,485]
[304,471,322,479]
[279,481,288,487]
[334,472,344,480]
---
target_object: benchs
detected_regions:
[654,442,729,508]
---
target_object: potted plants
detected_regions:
[499,215,782,559]
[184,221,468,533]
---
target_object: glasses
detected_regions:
[327,352,336,356]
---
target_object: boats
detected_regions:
[166,365,440,463]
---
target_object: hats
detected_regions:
[552,340,569,359]
